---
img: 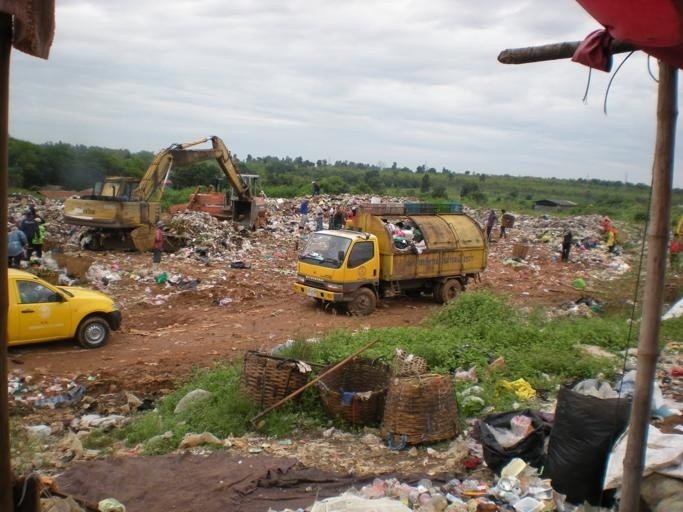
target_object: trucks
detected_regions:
[291,200,488,317]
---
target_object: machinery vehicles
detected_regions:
[169,174,267,222]
[61,135,258,252]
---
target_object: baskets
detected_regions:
[241,350,311,413]
[130,226,156,251]
[501,214,514,229]
[513,243,529,259]
[382,374,461,451]
[391,348,427,377]
[362,202,463,215]
[318,357,391,425]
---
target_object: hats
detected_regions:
[157,221,166,229]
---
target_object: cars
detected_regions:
[6,269,121,348]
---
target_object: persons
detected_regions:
[30,217,45,258]
[560,229,572,263]
[485,209,497,239]
[28,206,45,225]
[497,208,507,239]
[152,222,165,263]
[310,180,320,197]
[668,234,682,274]
[7,225,28,269]
[257,190,267,199]
[18,208,37,261]
[298,197,354,232]
